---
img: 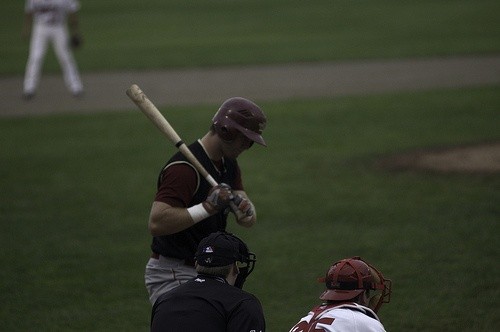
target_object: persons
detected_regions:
[151,231,266,332]
[20,0,83,100]
[289,256,392,332]
[144,97,268,307]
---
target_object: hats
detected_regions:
[197,231,249,266]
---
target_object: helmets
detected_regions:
[215,96,268,147]
[319,259,372,300]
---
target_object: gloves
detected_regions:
[202,183,234,216]
[233,194,256,228]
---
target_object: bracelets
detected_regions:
[186,203,211,224]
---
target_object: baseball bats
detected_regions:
[125,84,246,224]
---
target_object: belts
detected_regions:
[151,251,196,267]
